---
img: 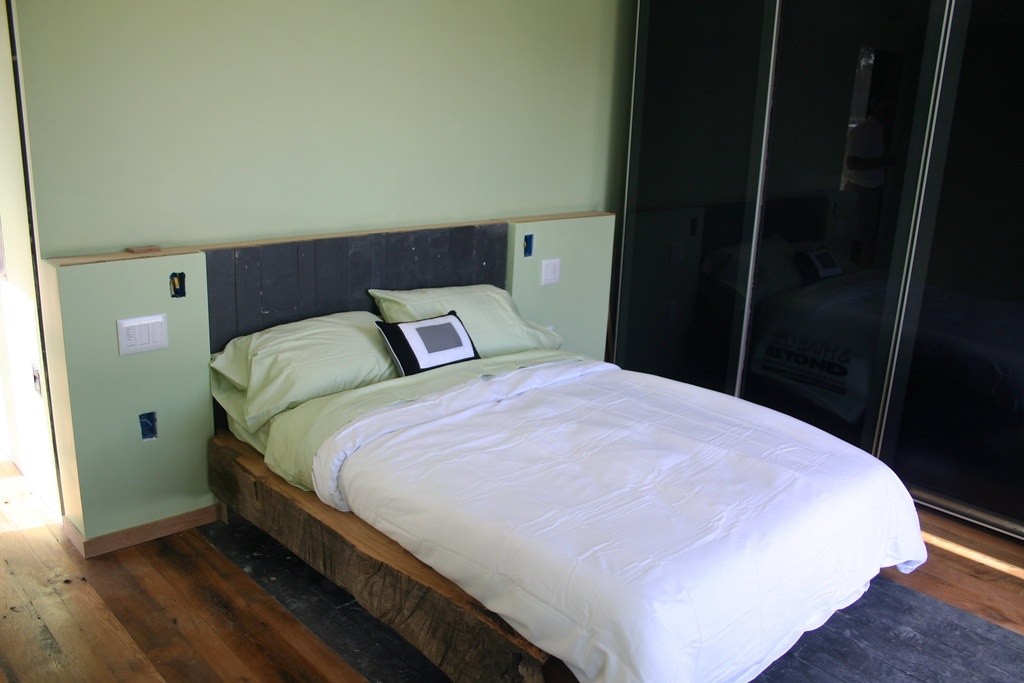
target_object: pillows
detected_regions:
[368,284,564,358]
[210,311,400,434]
[373,310,482,377]
[701,231,856,301]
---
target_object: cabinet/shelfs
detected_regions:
[613,0,950,457]
[875,0,1024,540]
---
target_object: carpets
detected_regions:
[197,512,1024,683]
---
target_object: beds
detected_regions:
[689,269,1024,483]
[207,349,928,683]
